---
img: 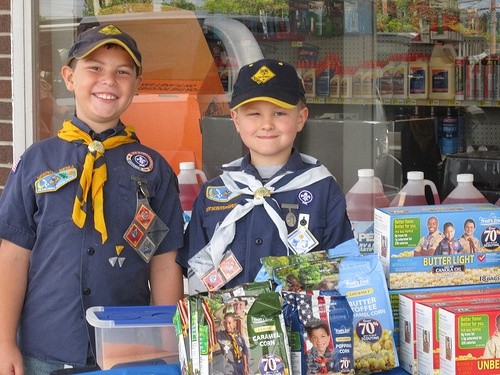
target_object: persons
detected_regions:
[413,216,480,256]
[175,59,354,296]
[212,276,341,375]
[0,22,185,375]
[482,314,500,359]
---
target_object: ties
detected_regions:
[423,234,432,250]
[208,164,335,269]
[59,120,139,245]
[317,358,330,374]
[228,333,241,363]
[462,235,476,254]
[446,238,455,255]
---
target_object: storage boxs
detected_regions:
[84,204,500,375]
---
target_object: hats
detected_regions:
[228,58,305,110]
[64,24,142,68]
